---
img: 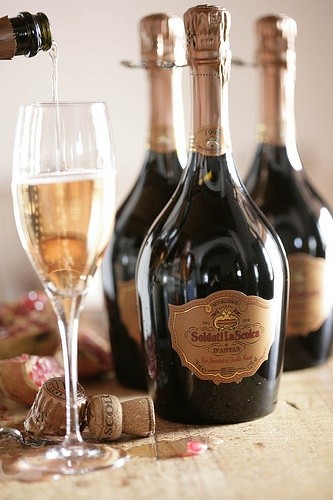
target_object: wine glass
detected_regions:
[13,100,127,476]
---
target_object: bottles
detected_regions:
[134,4,290,427]
[0,11,52,61]
[241,13,333,372]
[103,13,186,389]
